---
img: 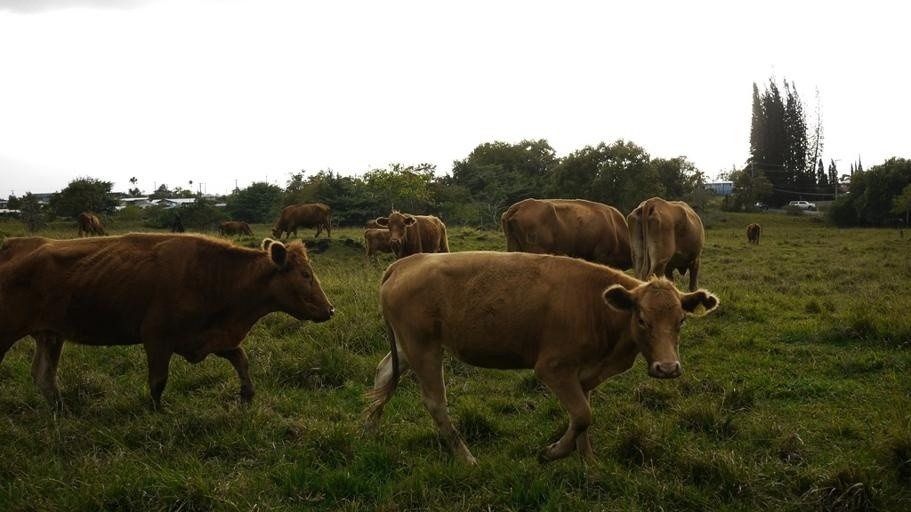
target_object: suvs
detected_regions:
[789,200,817,211]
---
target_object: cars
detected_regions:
[754,200,769,211]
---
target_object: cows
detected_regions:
[746,223,760,246]
[360,250,721,470]
[78,213,108,237]
[219,221,255,238]
[364,228,394,258]
[376,210,451,259]
[501,198,634,273]
[272,202,332,240]
[0,231,335,412]
[626,196,705,293]
